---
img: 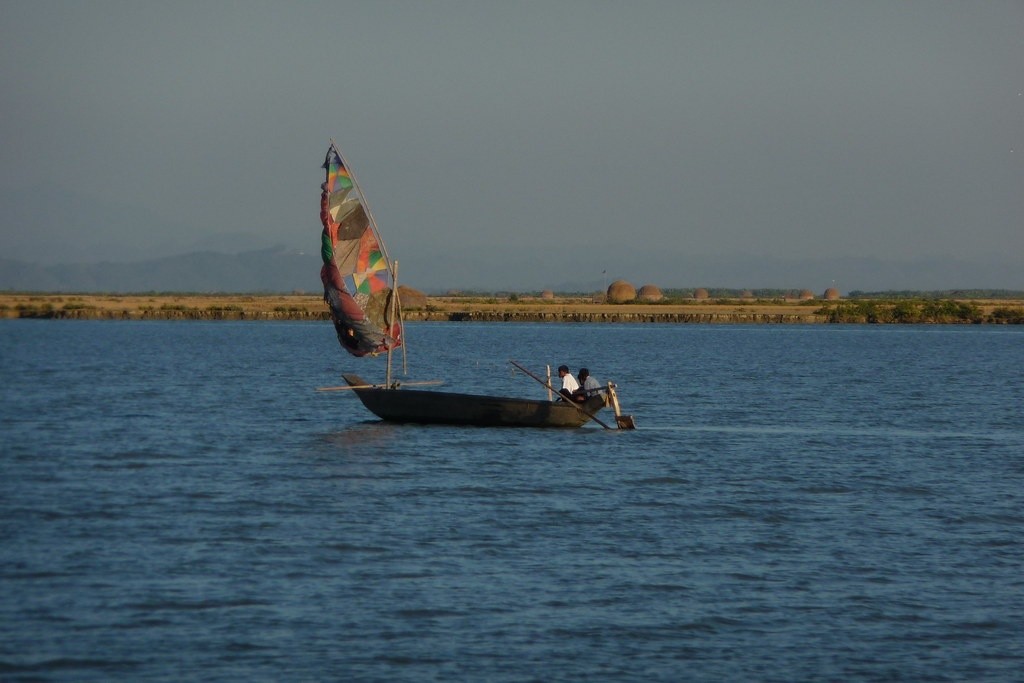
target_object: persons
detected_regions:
[572,368,602,397]
[558,365,579,395]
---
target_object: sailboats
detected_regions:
[319,140,635,428]
